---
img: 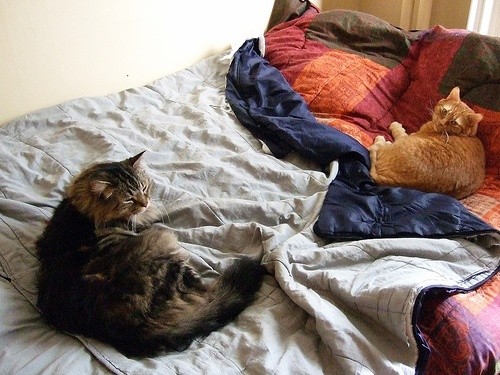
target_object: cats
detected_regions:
[37,150,264,358]
[369,87,487,199]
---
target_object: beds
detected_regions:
[0,45,500,375]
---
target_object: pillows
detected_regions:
[367,22,500,177]
[263,10,431,131]
